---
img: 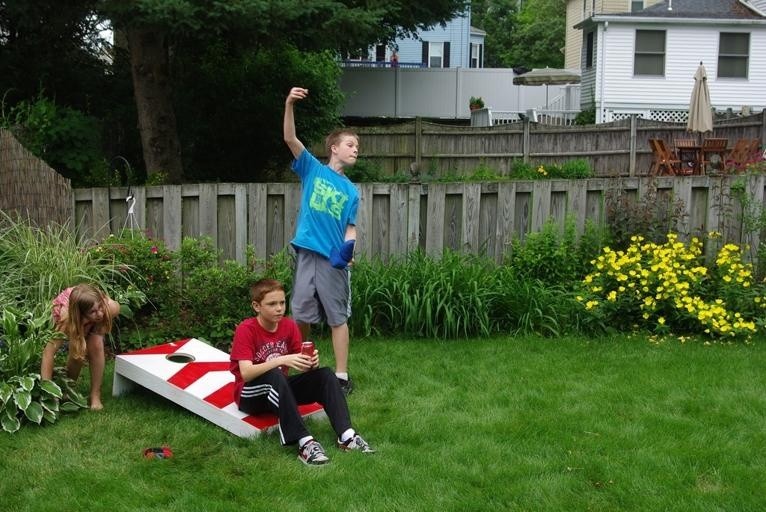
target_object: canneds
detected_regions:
[302,341,316,362]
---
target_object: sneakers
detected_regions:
[337,377,352,397]
[297,439,329,467]
[337,433,376,454]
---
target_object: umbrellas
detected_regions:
[688,62,715,140]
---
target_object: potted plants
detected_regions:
[469,96,484,111]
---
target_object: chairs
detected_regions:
[647,138,762,177]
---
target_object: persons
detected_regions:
[283,86,361,394]
[228,278,376,466]
[390,51,399,67]
[41,283,121,411]
